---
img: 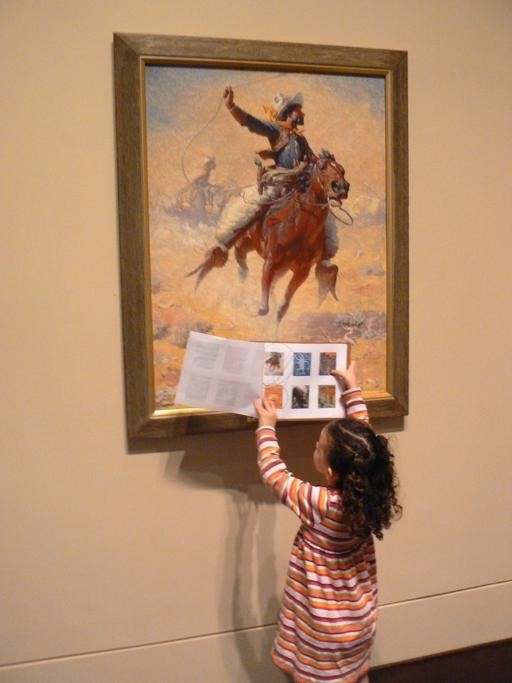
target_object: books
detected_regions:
[174,330,347,423]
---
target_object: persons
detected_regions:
[267,349,280,369]
[252,351,403,683]
[216,84,340,258]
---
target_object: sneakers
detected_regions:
[314,261,339,309]
[182,245,228,293]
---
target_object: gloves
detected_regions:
[223,86,249,127]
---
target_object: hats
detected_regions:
[273,91,304,121]
[200,154,215,168]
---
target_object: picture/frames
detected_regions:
[112,34,411,442]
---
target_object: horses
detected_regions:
[232,147,350,332]
